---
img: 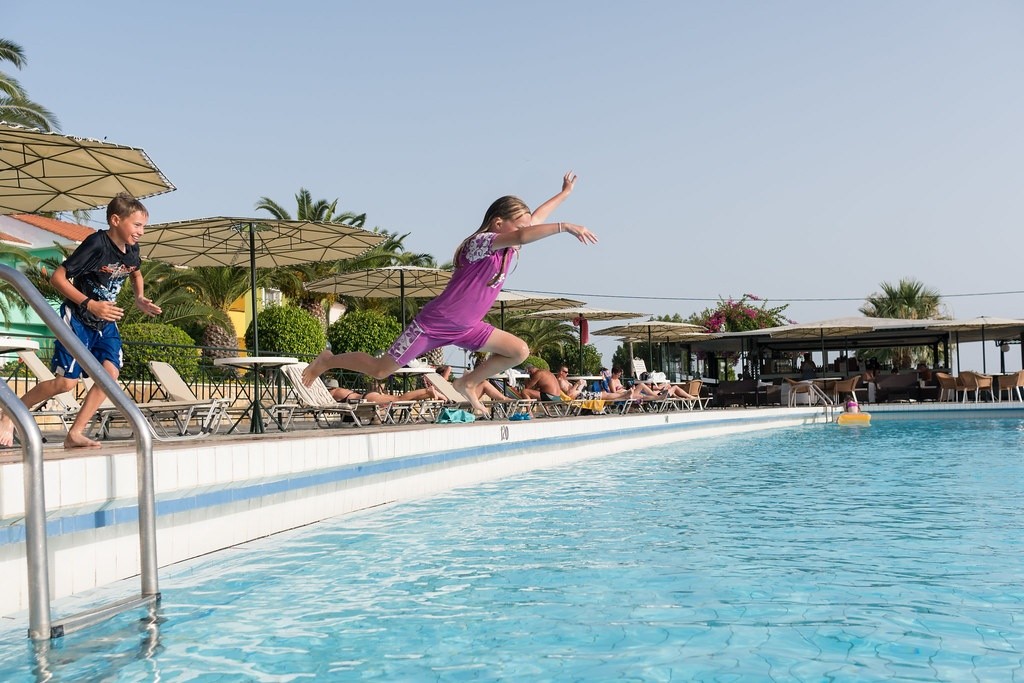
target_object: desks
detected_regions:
[982,373,1016,402]
[634,380,670,411]
[214,357,298,434]
[566,376,603,392]
[487,373,530,419]
[0,339,39,395]
[393,368,436,423]
[809,378,842,406]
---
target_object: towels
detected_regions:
[437,407,475,423]
[559,390,614,411]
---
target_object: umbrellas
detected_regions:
[302,265,453,425]
[138,216,394,434]
[611,332,723,382]
[771,321,876,406]
[589,321,708,389]
[0,121,177,215]
[926,315,1024,402]
[509,308,654,378]
[485,289,589,415]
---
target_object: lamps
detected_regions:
[1001,343,1009,352]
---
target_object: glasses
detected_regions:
[563,371,567,373]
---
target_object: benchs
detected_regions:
[718,381,758,408]
[875,373,921,403]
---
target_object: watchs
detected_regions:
[80,297,91,310]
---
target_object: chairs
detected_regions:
[936,370,1024,403]
[783,375,861,406]
[263,361,713,432]
[16,351,241,447]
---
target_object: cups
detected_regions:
[581,391,602,400]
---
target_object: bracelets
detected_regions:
[558,222,562,233]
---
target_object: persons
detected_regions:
[434,364,513,400]
[0,192,164,448]
[327,383,449,404]
[558,365,700,399]
[800,351,817,372]
[522,366,560,419]
[742,364,752,379]
[301,169,599,413]
